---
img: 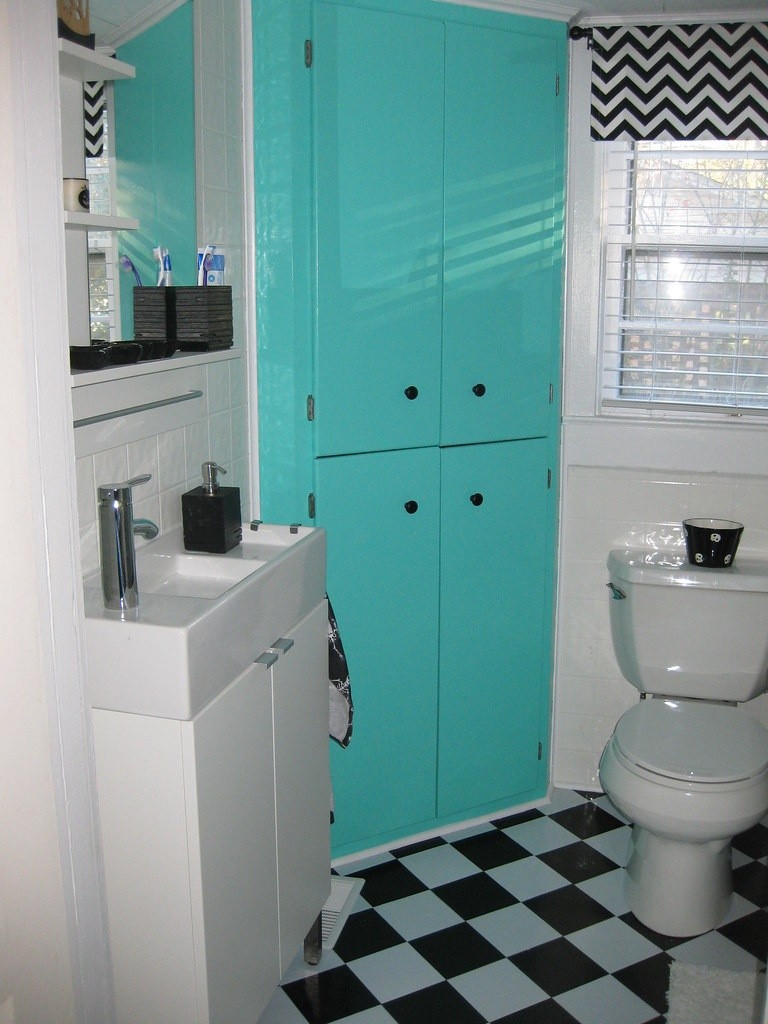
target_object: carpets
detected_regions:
[667,958,768,1024]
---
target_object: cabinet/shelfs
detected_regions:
[88,599,331,1024]
[257,437,561,848]
[255,4,554,459]
[57,0,139,231]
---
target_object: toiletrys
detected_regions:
[181,461,243,554]
[197,243,225,286]
[157,246,173,287]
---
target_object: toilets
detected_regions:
[597,550,768,939]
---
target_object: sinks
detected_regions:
[81,544,264,628]
[188,527,315,562]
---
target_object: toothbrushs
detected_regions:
[153,244,165,286]
[197,244,217,287]
[203,252,215,287]
[119,254,142,286]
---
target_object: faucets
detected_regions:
[96,473,157,610]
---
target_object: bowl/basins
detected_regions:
[71,346,112,370]
[133,340,160,360]
[110,343,142,364]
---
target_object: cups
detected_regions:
[134,286,176,356]
[682,518,745,568]
[178,284,234,350]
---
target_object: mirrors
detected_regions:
[81,1,196,348]
